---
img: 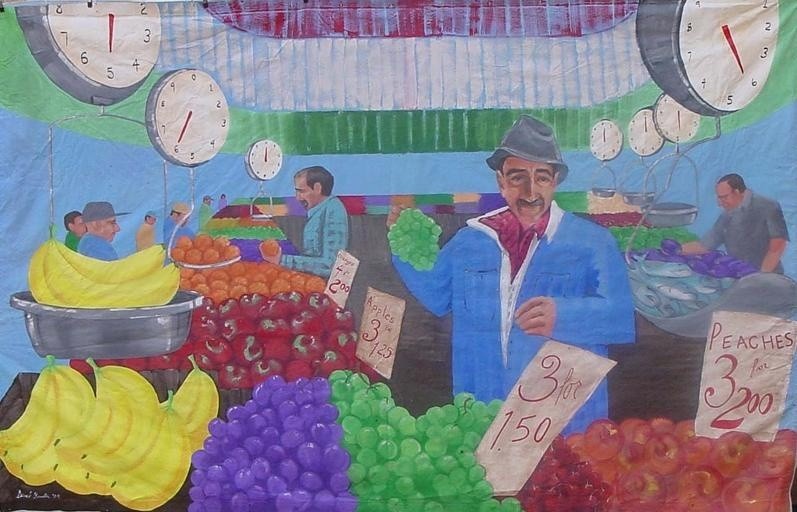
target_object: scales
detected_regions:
[11,5,289,362]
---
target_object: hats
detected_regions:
[486,114,568,184]
[171,203,189,214]
[78,201,129,225]
[204,195,213,201]
[146,211,158,218]
